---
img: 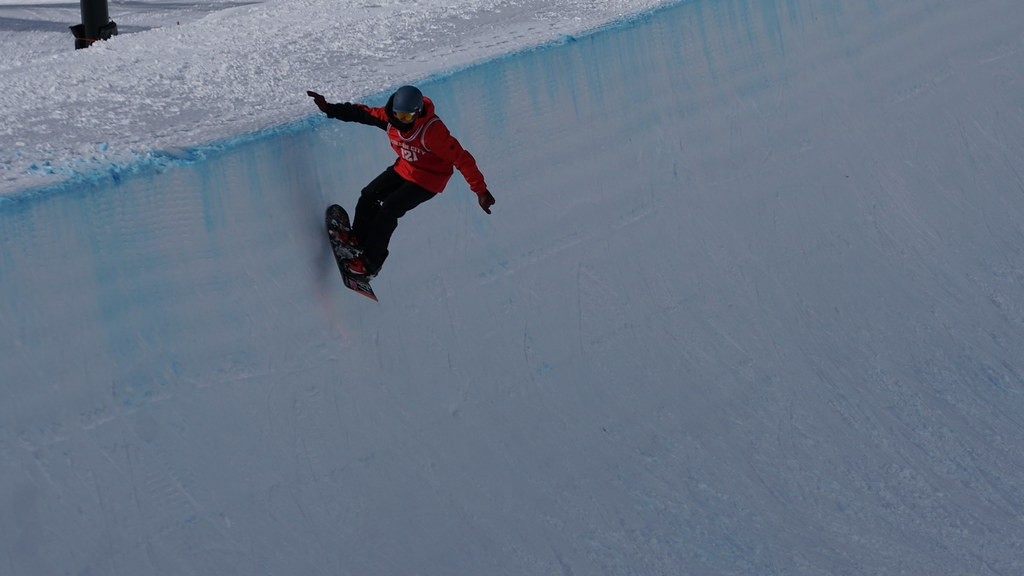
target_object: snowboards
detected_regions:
[325,203,379,303]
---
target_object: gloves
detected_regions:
[307,91,329,115]
[478,190,495,214]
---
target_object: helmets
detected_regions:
[392,86,424,113]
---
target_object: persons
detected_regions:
[307,86,495,280]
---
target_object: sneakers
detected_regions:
[329,222,363,255]
[340,255,370,275]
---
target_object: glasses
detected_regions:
[394,112,416,121]
